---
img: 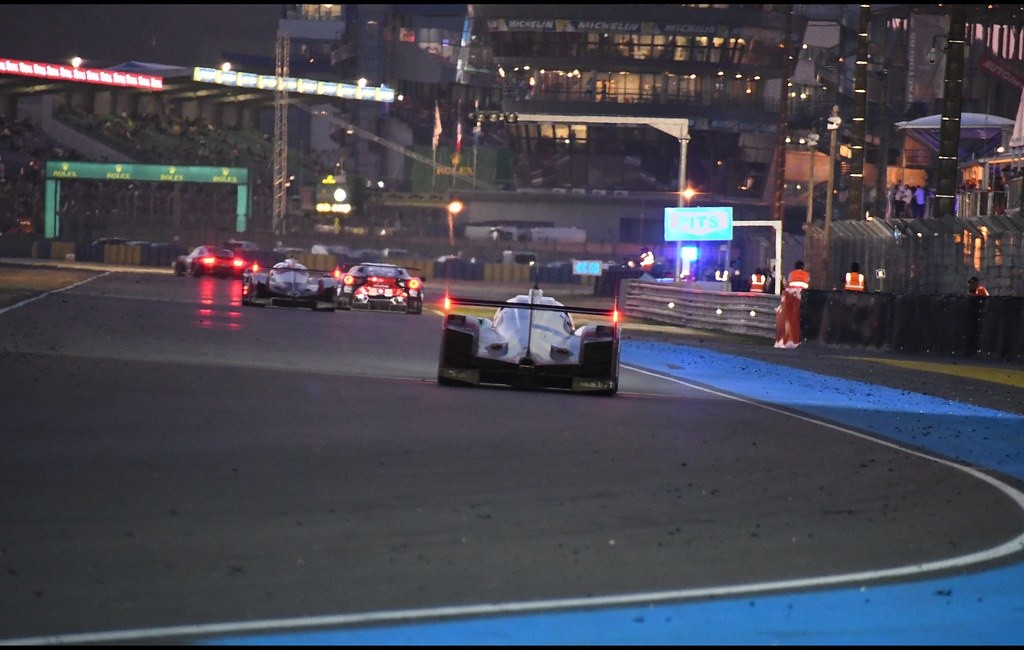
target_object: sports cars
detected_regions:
[174,244,245,277]
[243,258,339,311]
[338,263,424,314]
[438,282,622,398]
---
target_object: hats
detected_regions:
[967,277,980,283]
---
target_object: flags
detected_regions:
[457,121,461,152]
[475,128,479,155]
[433,106,442,148]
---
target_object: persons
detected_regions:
[636,247,656,273]
[842,262,869,292]
[748,266,786,294]
[891,179,925,218]
[788,262,810,288]
[0,101,325,225]
[708,255,742,281]
[967,276,989,297]
